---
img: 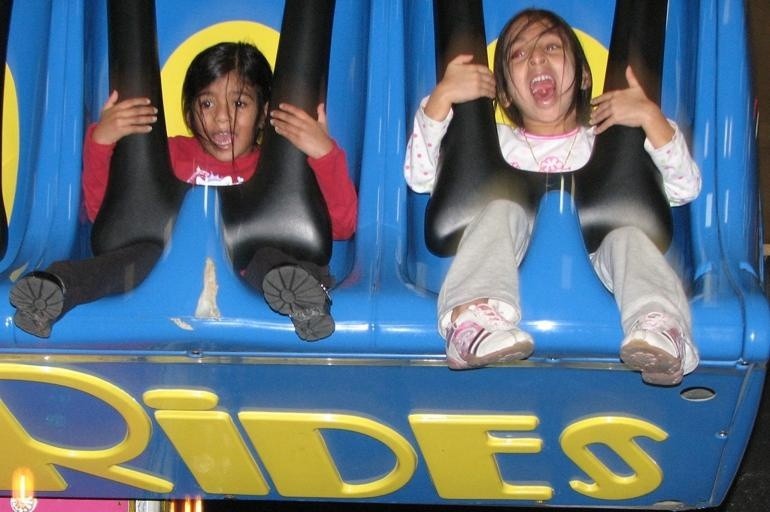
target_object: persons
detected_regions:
[9,40,358,341]
[402,7,703,386]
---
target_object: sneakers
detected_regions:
[259,263,336,342]
[445,304,535,370]
[619,311,685,387]
[8,270,66,338]
